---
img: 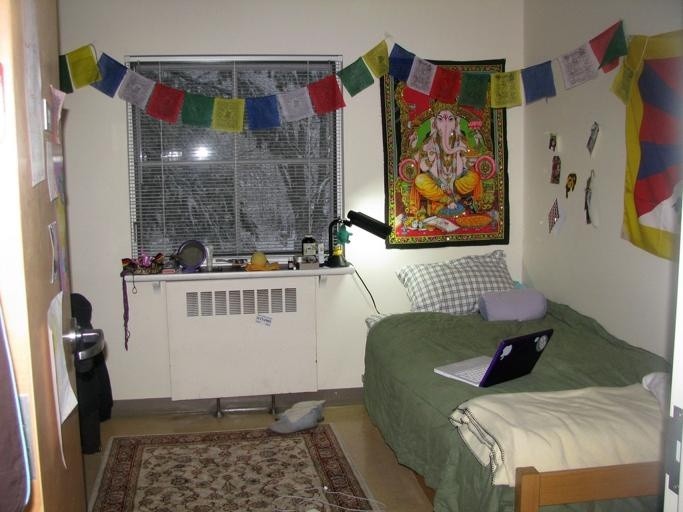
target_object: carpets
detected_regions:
[84,423,378,511]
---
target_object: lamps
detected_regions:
[323,210,392,268]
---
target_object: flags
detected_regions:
[181,93,282,132]
[621,28,678,264]
[278,39,391,123]
[52,39,184,123]
[388,19,629,111]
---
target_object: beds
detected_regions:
[357,283,672,512]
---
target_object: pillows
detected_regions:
[478,286,547,323]
[395,251,516,314]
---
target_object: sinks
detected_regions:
[78,329,104,360]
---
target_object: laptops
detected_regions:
[433,328,553,388]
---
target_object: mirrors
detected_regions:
[176,239,207,273]
